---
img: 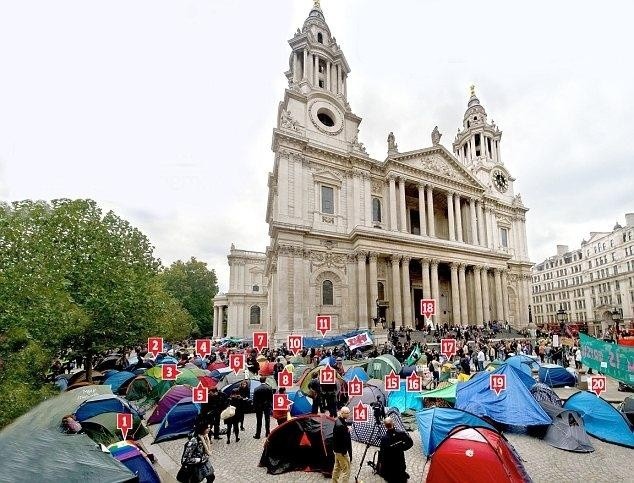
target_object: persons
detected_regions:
[387,132,396,149]
[431,126,442,146]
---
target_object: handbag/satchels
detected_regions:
[177,467,201,482]
[220,406,237,420]
[309,388,318,399]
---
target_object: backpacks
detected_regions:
[429,362,435,372]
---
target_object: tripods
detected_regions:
[355,420,385,483]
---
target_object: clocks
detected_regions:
[489,167,510,194]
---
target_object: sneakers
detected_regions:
[240,425,244,430]
[253,433,260,439]
[215,435,240,443]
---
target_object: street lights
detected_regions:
[612,306,622,335]
[556,308,569,334]
